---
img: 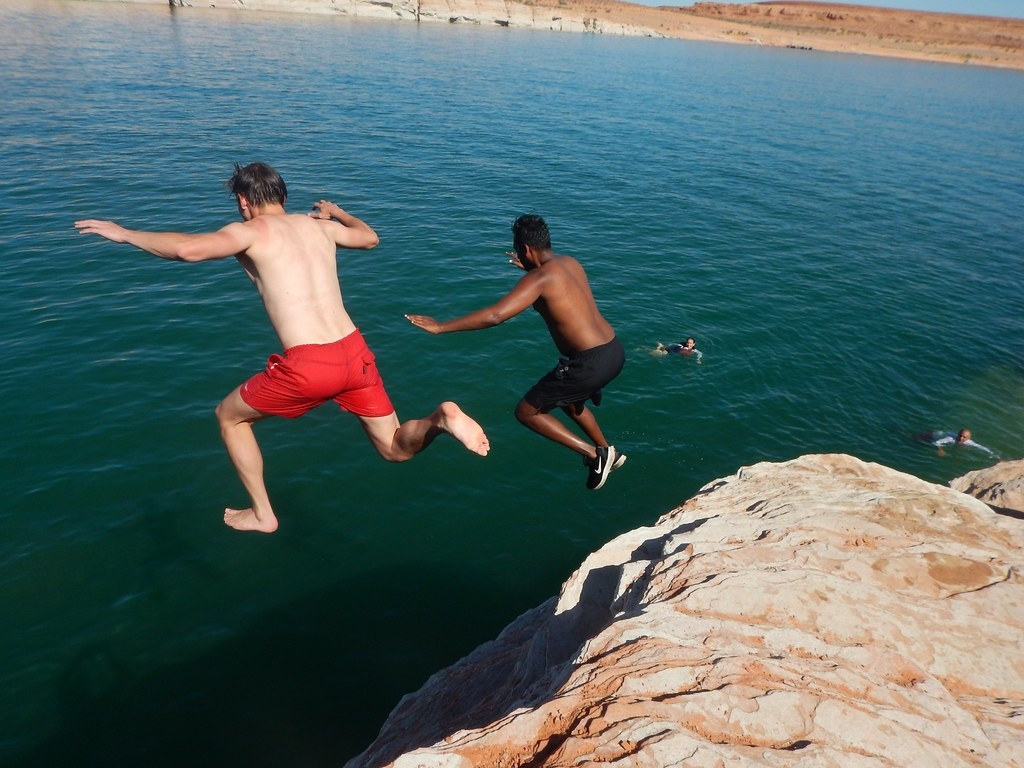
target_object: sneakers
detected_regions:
[609,453,626,474]
[582,446,615,489]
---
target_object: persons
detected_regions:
[404,214,626,489]
[939,428,970,442]
[75,162,490,533]
[676,337,696,351]
